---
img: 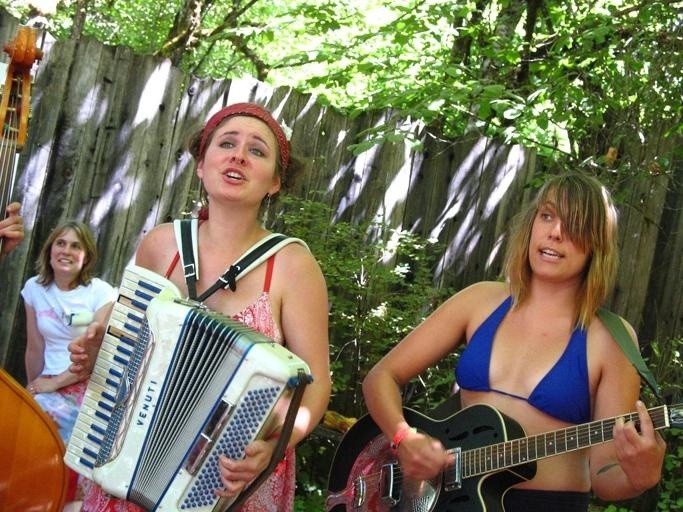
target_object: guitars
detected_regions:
[323,401,681,511]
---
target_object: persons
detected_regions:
[361,175,669,512]
[20,222,116,448]
[82,103,329,511]
[1,200,25,259]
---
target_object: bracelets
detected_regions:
[390,426,415,456]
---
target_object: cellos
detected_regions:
[0,22,71,512]
